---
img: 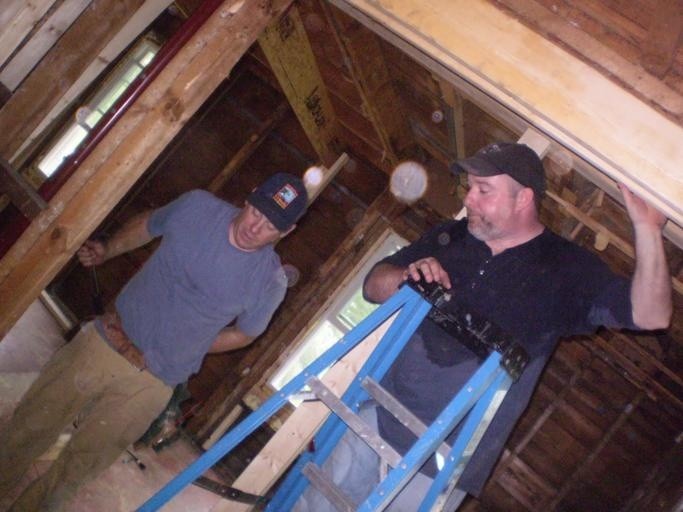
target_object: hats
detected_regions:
[247,173,307,232]
[447,143,546,199]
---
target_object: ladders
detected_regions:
[134,269,533,512]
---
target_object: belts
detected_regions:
[100,311,147,371]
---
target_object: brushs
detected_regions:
[127,450,146,471]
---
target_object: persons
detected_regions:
[1,171,308,509]
[296,138,672,512]
